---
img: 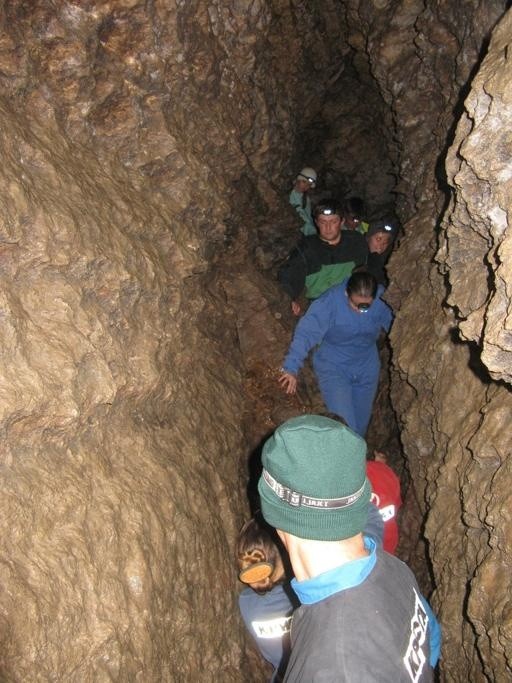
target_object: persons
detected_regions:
[362,209,399,268]
[288,167,318,235]
[234,514,300,682]
[277,271,394,437]
[339,195,369,235]
[366,447,402,554]
[256,413,441,682]
[281,199,369,317]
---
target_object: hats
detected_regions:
[254,412,381,546]
[296,166,317,189]
